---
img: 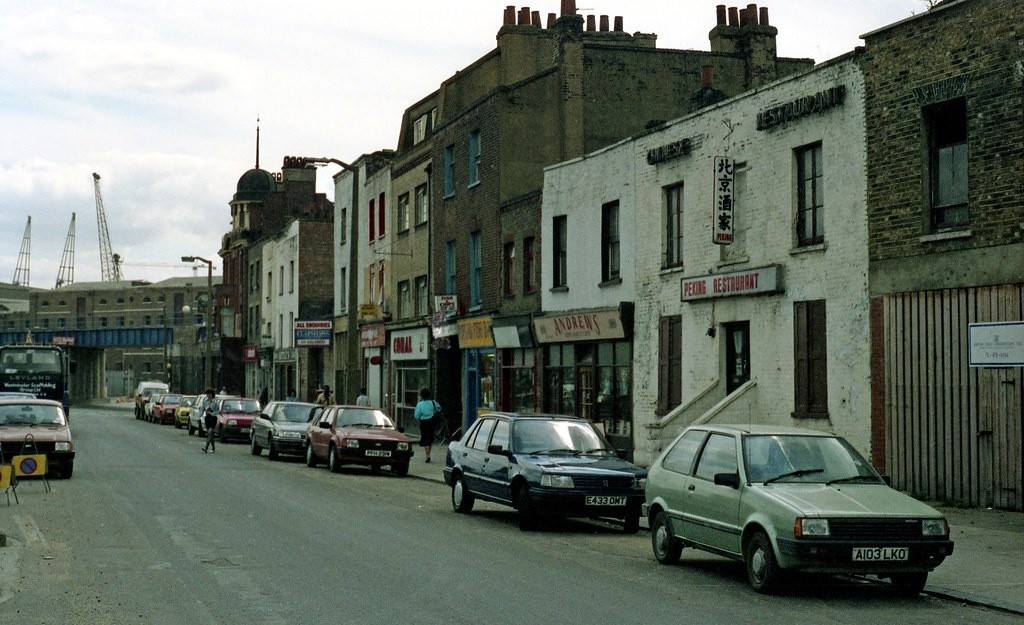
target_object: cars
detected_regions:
[175,395,197,428]
[305,405,414,477]
[143,394,162,423]
[151,395,181,425]
[643,425,956,596]
[443,412,647,537]
[0,399,75,478]
[250,400,323,462]
[189,394,235,438]
[215,396,262,444]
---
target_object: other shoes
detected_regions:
[201,448,216,453]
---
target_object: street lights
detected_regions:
[181,256,213,390]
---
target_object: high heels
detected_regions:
[425,457,431,463]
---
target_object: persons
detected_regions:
[285,389,300,402]
[196,388,219,453]
[357,388,372,407]
[219,386,226,395]
[135,394,145,421]
[316,385,337,405]
[259,384,269,405]
[414,388,443,463]
[3,355,14,364]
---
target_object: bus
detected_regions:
[0,346,70,422]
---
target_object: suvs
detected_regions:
[135,387,167,421]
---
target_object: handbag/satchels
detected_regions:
[431,400,445,429]
